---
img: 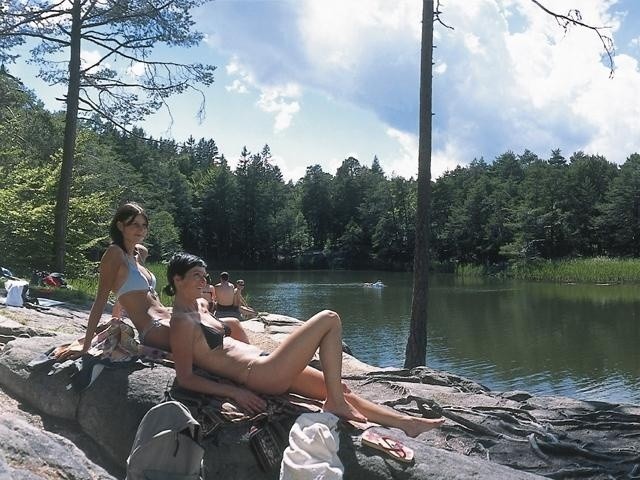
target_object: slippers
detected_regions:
[360,428,415,463]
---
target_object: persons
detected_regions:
[56,202,170,362]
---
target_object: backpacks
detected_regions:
[124,401,206,480]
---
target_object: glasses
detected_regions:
[238,284,244,286]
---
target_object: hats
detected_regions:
[50,272,60,278]
